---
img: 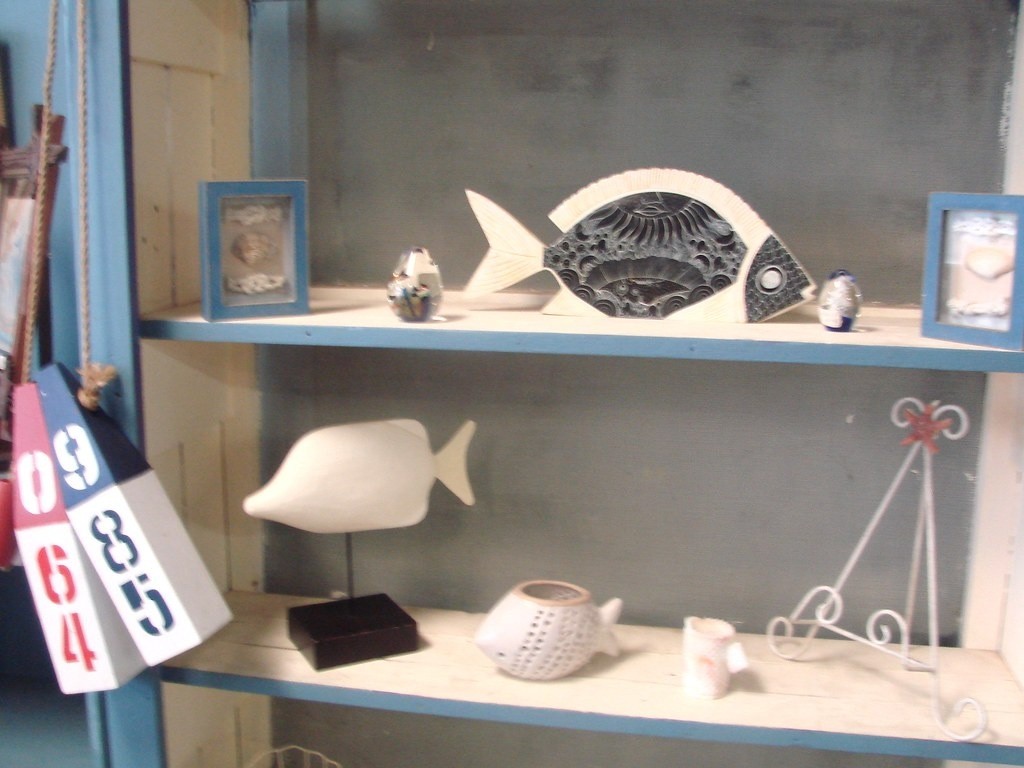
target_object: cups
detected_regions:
[681,615,735,700]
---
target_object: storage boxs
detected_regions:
[283,590,419,670]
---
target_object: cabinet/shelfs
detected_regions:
[121,0,1024,767]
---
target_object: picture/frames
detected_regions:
[196,180,310,321]
[922,193,1024,348]
